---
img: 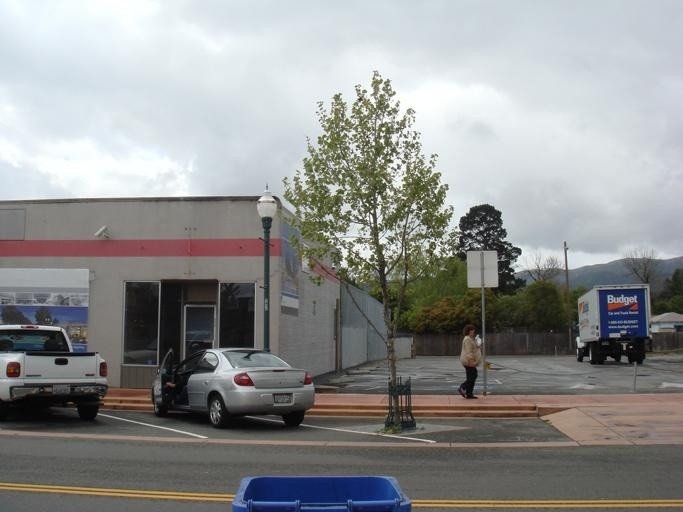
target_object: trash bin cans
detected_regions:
[232,475,411,512]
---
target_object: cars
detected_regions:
[150,346,315,429]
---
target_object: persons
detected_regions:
[165,381,188,405]
[456,325,482,399]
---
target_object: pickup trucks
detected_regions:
[0,324,108,420]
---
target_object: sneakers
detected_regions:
[458,388,477,399]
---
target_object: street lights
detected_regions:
[256,184,278,349]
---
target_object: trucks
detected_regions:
[574,283,650,365]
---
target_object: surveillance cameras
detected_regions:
[331,262,337,270]
[94,225,108,237]
